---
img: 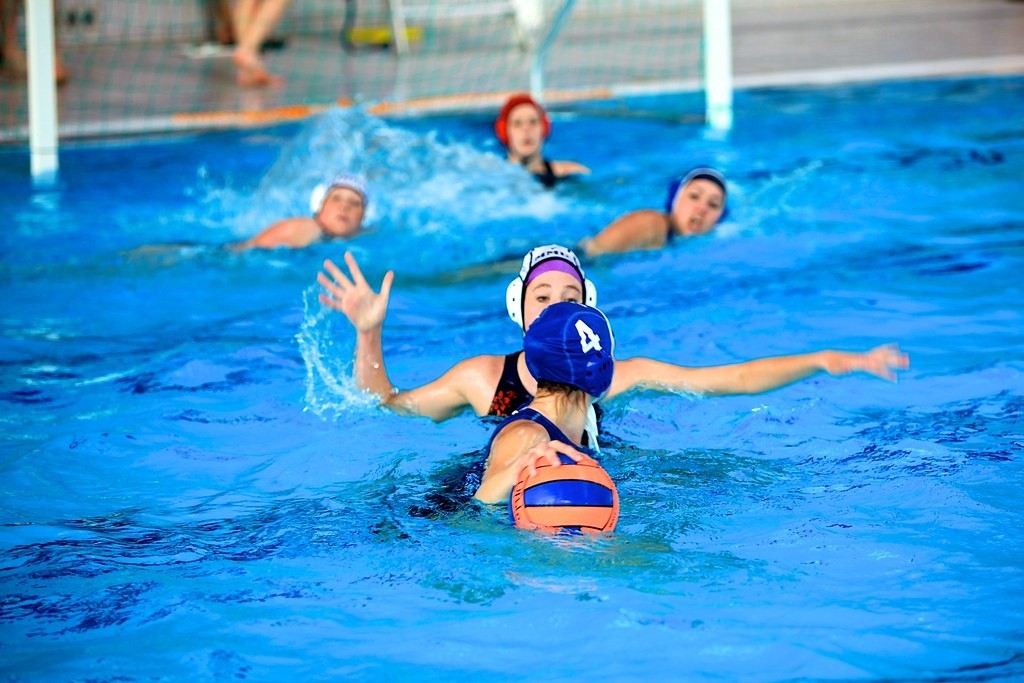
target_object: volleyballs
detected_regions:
[510,451,621,536]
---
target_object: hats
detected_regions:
[524,300,615,400]
[664,165,729,238]
[495,93,550,147]
[505,244,597,328]
[310,178,368,238]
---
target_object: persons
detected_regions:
[232,0,291,87]
[4,0,69,84]
[585,166,730,253]
[317,245,911,420]
[496,93,589,188]
[474,301,615,503]
[250,174,369,247]
